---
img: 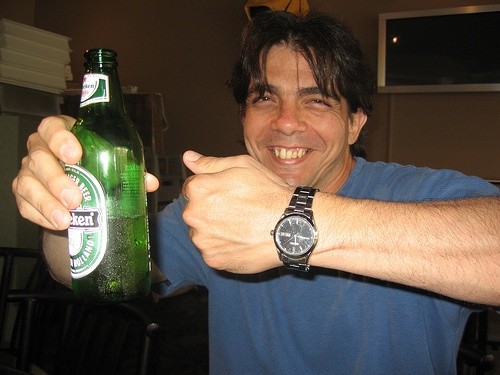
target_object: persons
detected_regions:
[11,12,500,375]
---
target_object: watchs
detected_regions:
[271,186,320,272]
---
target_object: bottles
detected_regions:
[63,48,152,302]
